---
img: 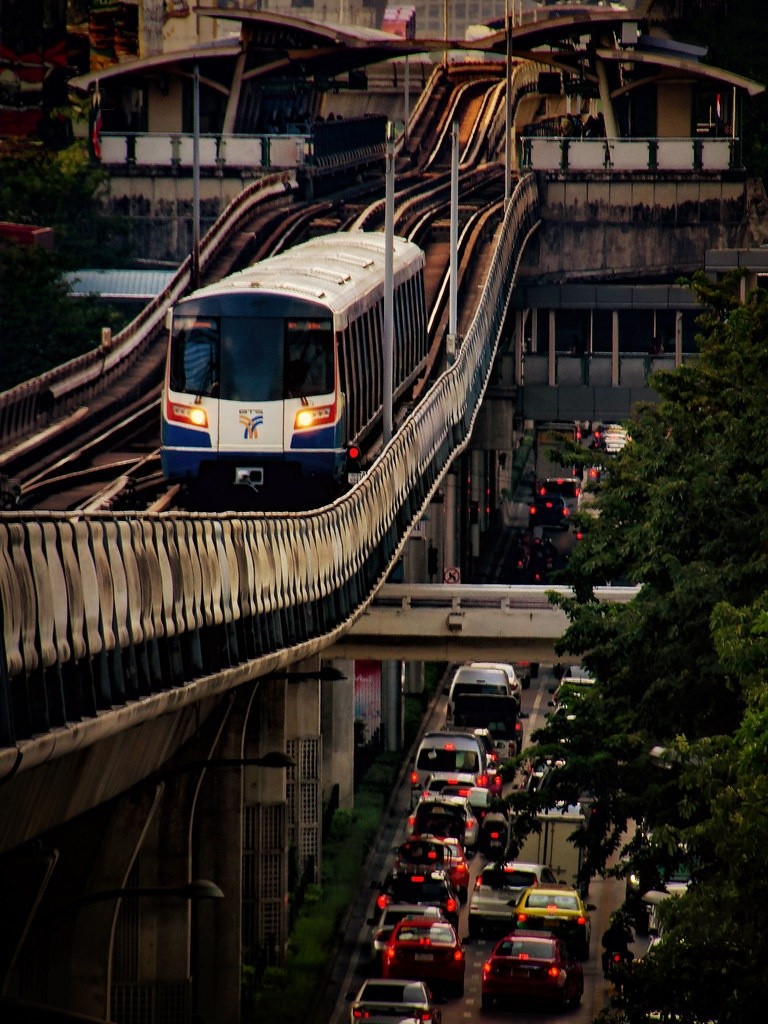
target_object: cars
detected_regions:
[513,883,597,962]
[627,824,704,929]
[468,859,567,940]
[375,915,468,999]
[369,658,609,925]
[366,904,449,971]
[528,494,566,532]
[345,976,442,1024]
[482,929,584,1012]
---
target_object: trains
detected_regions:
[159,228,429,508]
